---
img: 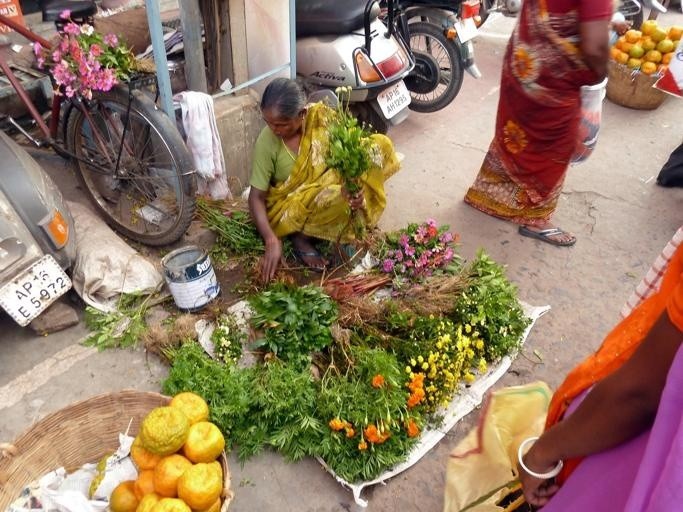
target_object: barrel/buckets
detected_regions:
[159,245,221,312]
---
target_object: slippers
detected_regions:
[519,226,576,246]
[297,249,325,273]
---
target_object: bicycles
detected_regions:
[0,1,199,245]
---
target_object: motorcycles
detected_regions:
[610,0,669,30]
[296,0,522,135]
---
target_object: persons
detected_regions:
[516,230,683,511]
[607,11,632,49]
[464,0,615,246]
[247,78,402,284]
[655,142,682,188]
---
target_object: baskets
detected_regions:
[606,58,668,110]
[0,389,234,512]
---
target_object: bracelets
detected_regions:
[518,438,564,480]
